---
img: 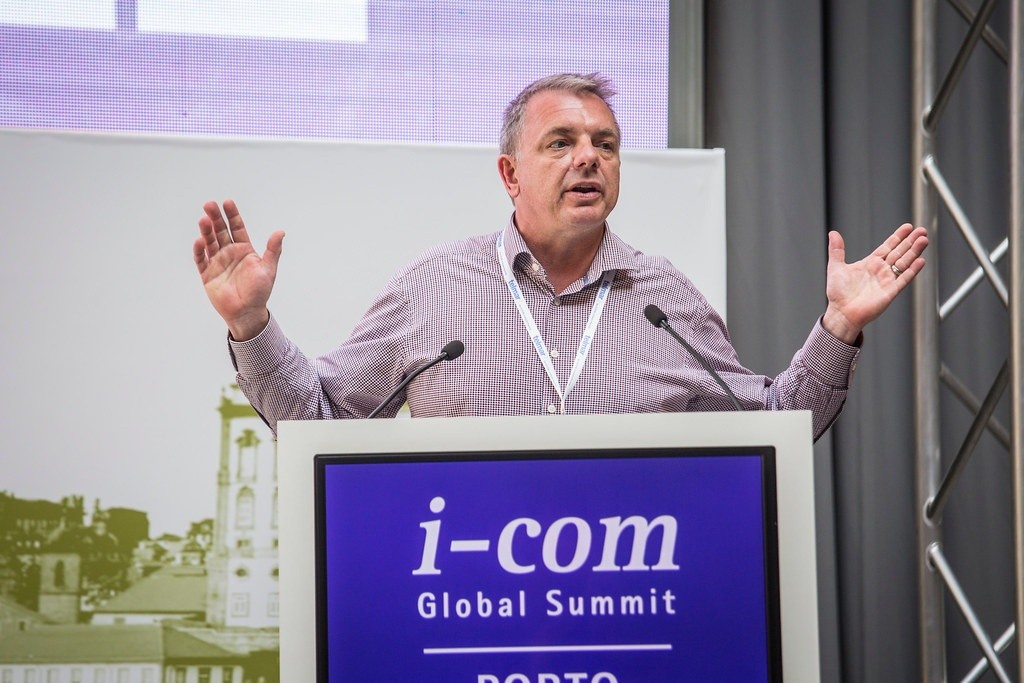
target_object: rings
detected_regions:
[891,265,900,275]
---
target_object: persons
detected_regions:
[193,72,929,444]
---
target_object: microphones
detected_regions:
[643,304,745,412]
[369,340,464,420]
